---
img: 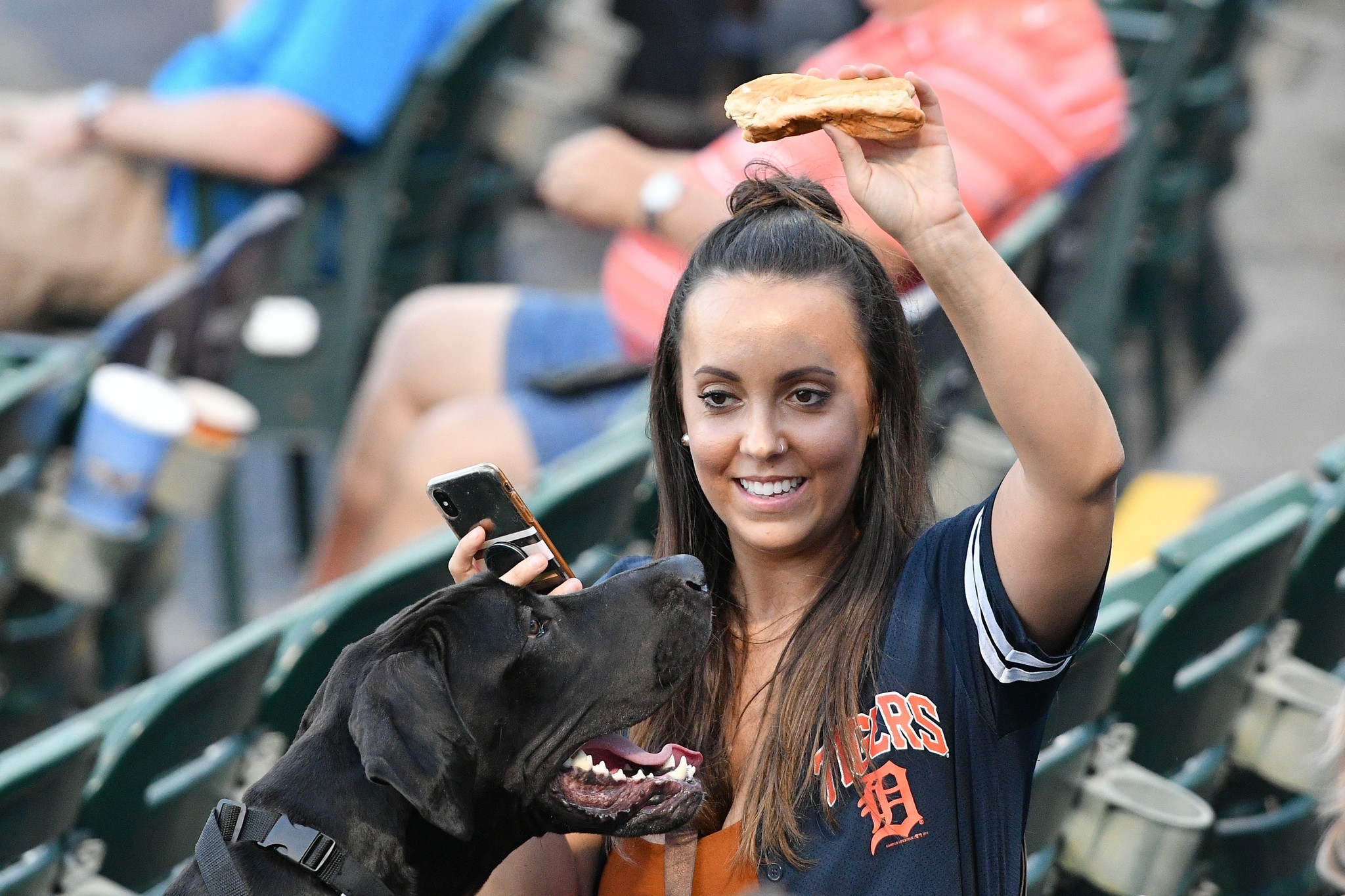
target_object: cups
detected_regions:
[180,378,259,456]
[67,362,191,528]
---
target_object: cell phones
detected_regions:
[426,463,577,594]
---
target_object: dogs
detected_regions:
[158,553,711,896]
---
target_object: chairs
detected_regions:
[0,0,1345,896]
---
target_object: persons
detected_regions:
[0,0,1134,896]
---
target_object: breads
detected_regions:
[724,72,925,140]
